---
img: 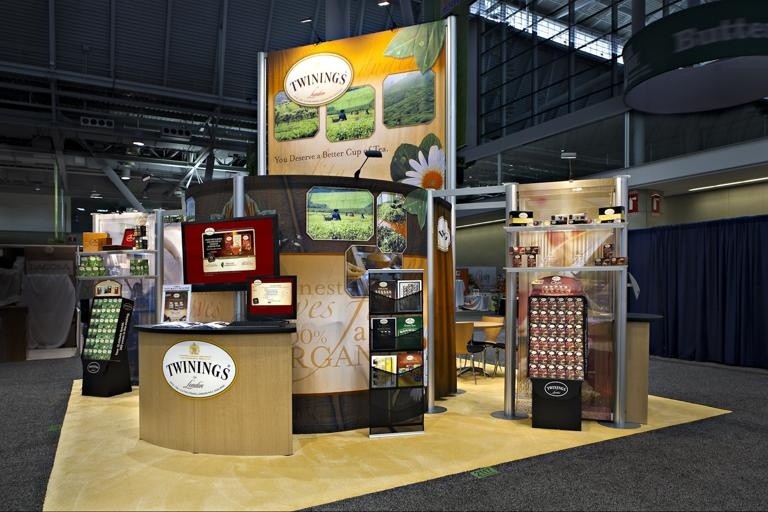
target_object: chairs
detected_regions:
[456,314,504,386]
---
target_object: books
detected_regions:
[370,317,396,351]
[398,279,422,312]
[371,280,396,313]
[397,316,422,350]
[371,355,397,388]
[397,353,422,386]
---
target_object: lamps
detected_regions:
[351,148,382,177]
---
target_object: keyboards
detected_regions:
[226,320,283,328]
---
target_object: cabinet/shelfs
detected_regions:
[505,184,662,428]
[74,243,162,395]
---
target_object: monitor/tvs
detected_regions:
[180,214,280,293]
[246,274,297,320]
